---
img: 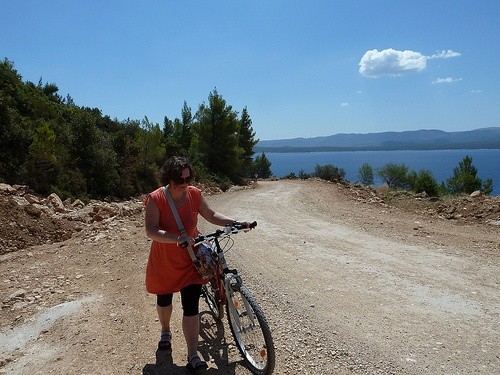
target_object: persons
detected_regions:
[144,156,255,369]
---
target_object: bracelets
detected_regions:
[177,235,181,247]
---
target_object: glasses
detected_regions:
[171,176,193,185]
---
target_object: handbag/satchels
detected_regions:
[192,242,224,284]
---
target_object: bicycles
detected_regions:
[176,221,276,375]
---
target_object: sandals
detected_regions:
[188,355,208,371]
[158,331,172,350]
